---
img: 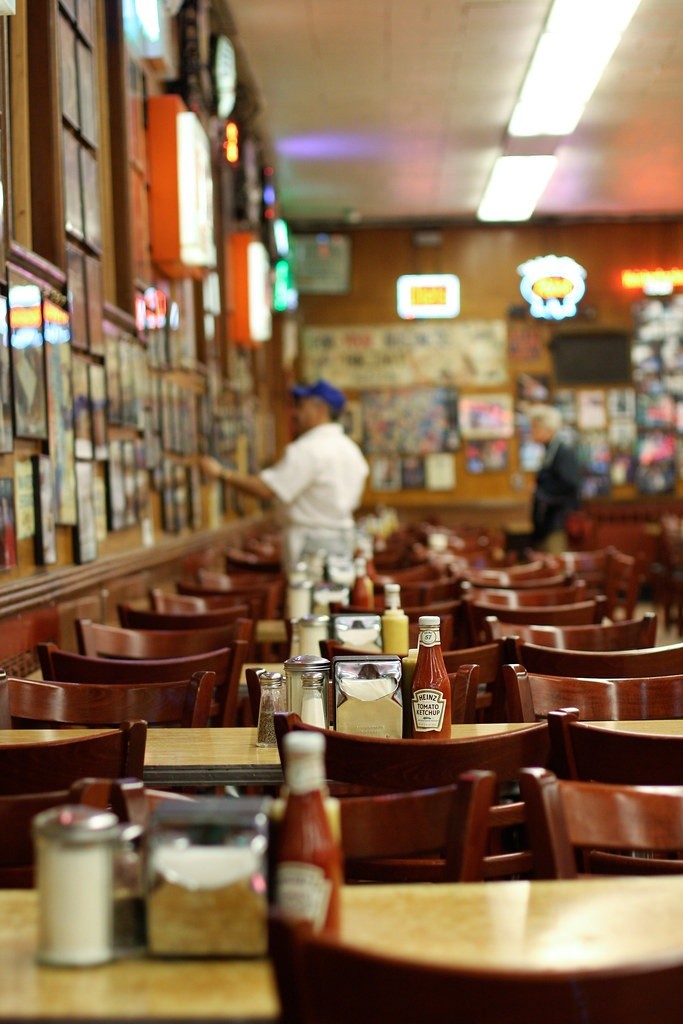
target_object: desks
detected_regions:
[1,874,681,1024]
[1,721,537,785]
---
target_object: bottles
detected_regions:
[255,670,283,747]
[300,671,327,728]
[32,805,120,967]
[411,615,452,739]
[283,656,330,730]
[284,581,312,619]
[384,584,401,607]
[300,614,330,657]
[290,618,300,657]
[364,544,377,581]
[382,605,409,656]
[268,732,340,955]
[354,556,374,610]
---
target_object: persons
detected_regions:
[528,438,611,547]
[190,379,372,579]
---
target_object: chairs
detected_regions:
[1,503,682,1024]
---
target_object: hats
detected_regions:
[293,379,344,408]
